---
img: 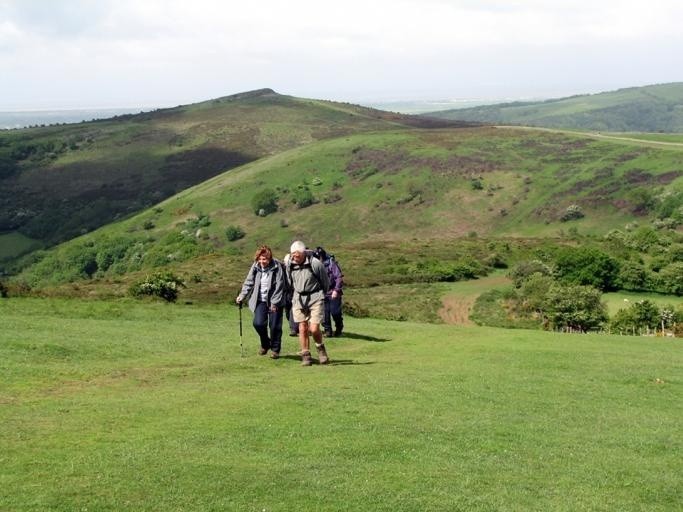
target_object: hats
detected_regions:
[291,241,305,253]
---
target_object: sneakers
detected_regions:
[289,331,298,336]
[326,330,333,337]
[334,323,343,337]
[259,341,272,355]
[271,352,279,360]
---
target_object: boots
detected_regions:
[302,349,312,366]
[315,343,329,364]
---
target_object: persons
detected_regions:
[283,254,299,337]
[282,241,330,367]
[236,245,284,359]
[312,247,344,338]
